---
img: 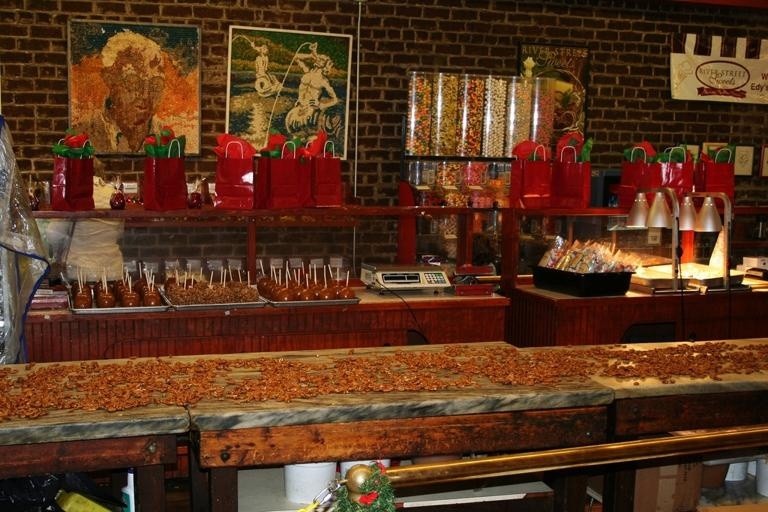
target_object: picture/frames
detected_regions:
[732,143,768,179]
[66,18,202,157]
[516,41,592,148]
[226,25,354,162]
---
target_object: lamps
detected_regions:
[622,186,753,295]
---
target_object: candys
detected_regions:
[540,239,637,273]
[405,71,555,209]
[73,260,356,308]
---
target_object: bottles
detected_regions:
[405,71,556,260]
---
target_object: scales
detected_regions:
[360,261,452,296]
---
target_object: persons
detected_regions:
[71,33,172,153]
[254,43,269,78]
[286,43,339,127]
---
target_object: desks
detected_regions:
[17,283,513,362]
[508,282,767,348]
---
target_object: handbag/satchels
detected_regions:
[510,146,736,211]
[142,140,340,210]
[51,135,93,211]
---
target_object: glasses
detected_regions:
[123,74,166,93]
